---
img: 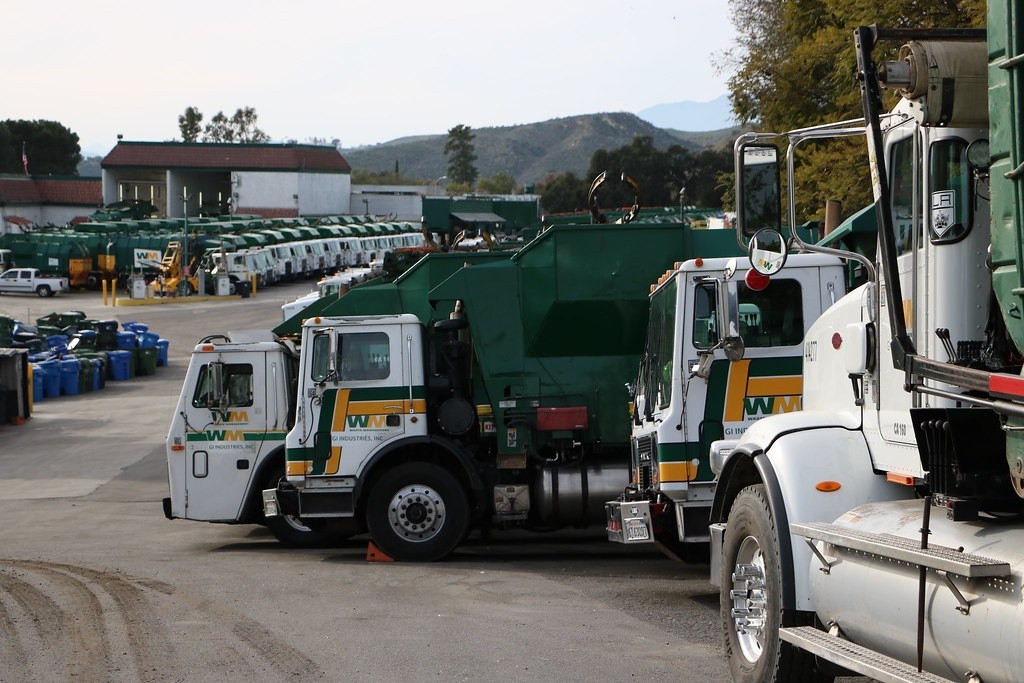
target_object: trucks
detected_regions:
[0,198,542,325]
[162,252,518,525]
[706,0,1024,681]
[261,222,824,565]
[604,200,879,567]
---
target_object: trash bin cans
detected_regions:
[0,310,170,422]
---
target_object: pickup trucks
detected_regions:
[0,267,69,298]
